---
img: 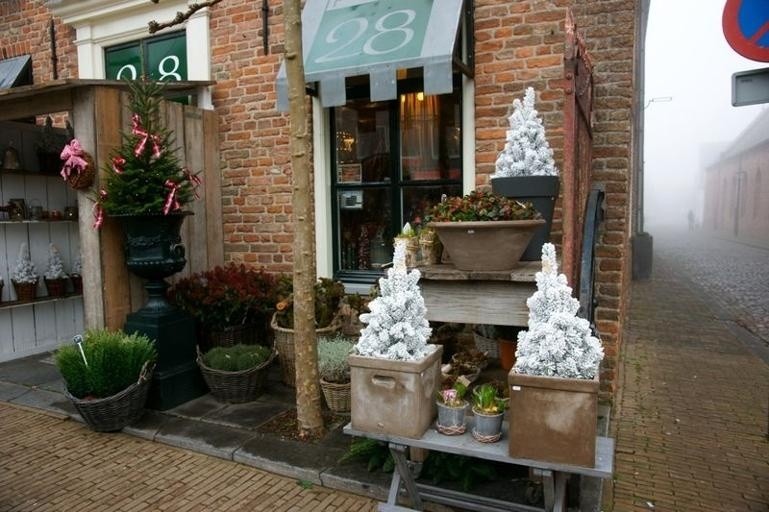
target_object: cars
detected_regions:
[358,151,413,198]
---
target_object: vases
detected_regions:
[425,220,545,269]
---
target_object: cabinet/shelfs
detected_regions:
[0,168,83,310]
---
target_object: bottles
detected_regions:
[358,224,370,270]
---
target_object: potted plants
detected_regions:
[347,242,444,440]
[508,243,604,470]
[491,86,559,260]
[495,325,528,370]
[473,325,500,358]
[418,227,443,265]
[470,383,506,444]
[393,229,419,267]
[433,384,468,436]
[317,332,358,413]
[89,36,204,315]
[270,277,346,389]
[0,243,82,305]
[55,327,158,434]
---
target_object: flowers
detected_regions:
[166,262,276,325]
[427,186,540,221]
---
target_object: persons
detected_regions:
[687,210,695,231]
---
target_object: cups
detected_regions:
[31,206,43,221]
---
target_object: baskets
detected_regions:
[319,377,351,412]
[58,361,157,432]
[65,152,95,190]
[270,310,341,388]
[202,319,253,348]
[11,276,40,301]
[471,324,501,363]
[452,332,473,353]
[43,276,68,296]
[70,273,83,295]
[369,238,394,270]
[36,148,61,177]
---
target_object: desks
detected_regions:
[383,260,560,462]
[341,414,614,512]
